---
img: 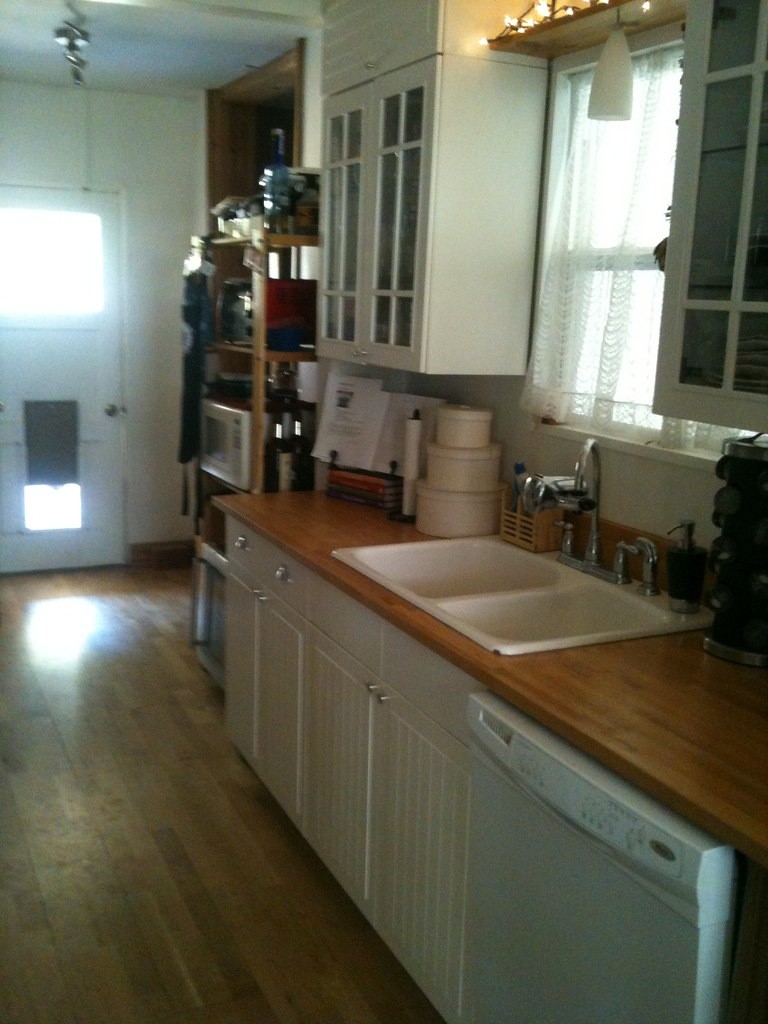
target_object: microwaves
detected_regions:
[199,392,276,490]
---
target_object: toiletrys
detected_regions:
[664,518,708,615]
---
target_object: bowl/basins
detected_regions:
[415,478,508,539]
[436,404,494,448]
[427,443,502,492]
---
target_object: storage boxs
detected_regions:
[499,491,564,554]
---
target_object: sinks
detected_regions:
[329,536,567,601]
[434,578,717,656]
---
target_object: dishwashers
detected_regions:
[464,690,736,1024]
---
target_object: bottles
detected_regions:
[263,129,290,234]
[290,415,311,491]
[266,414,292,493]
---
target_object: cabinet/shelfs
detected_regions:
[315,55,548,377]
[222,514,473,1024]
[191,233,320,696]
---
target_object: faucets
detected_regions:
[633,534,662,598]
[522,436,604,578]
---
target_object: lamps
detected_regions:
[588,8,633,121]
